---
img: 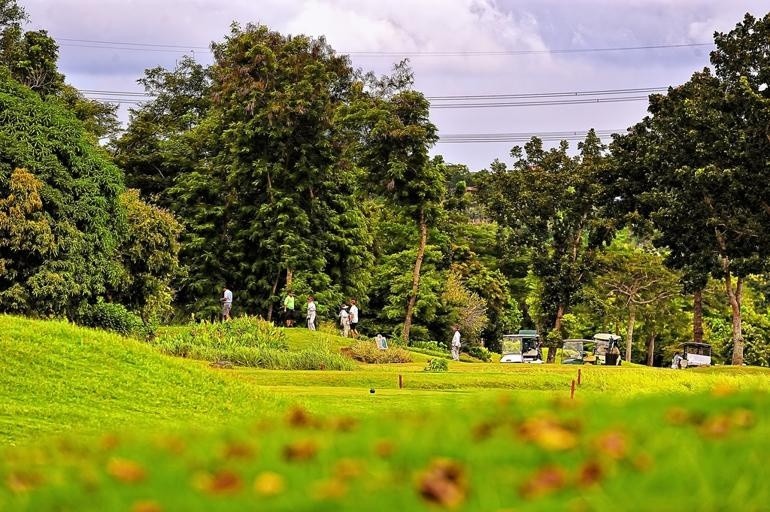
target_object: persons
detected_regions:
[307,296,317,330]
[451,327,461,362]
[349,300,359,337]
[671,351,682,370]
[612,343,621,366]
[339,304,350,337]
[284,289,295,327]
[220,286,233,323]
